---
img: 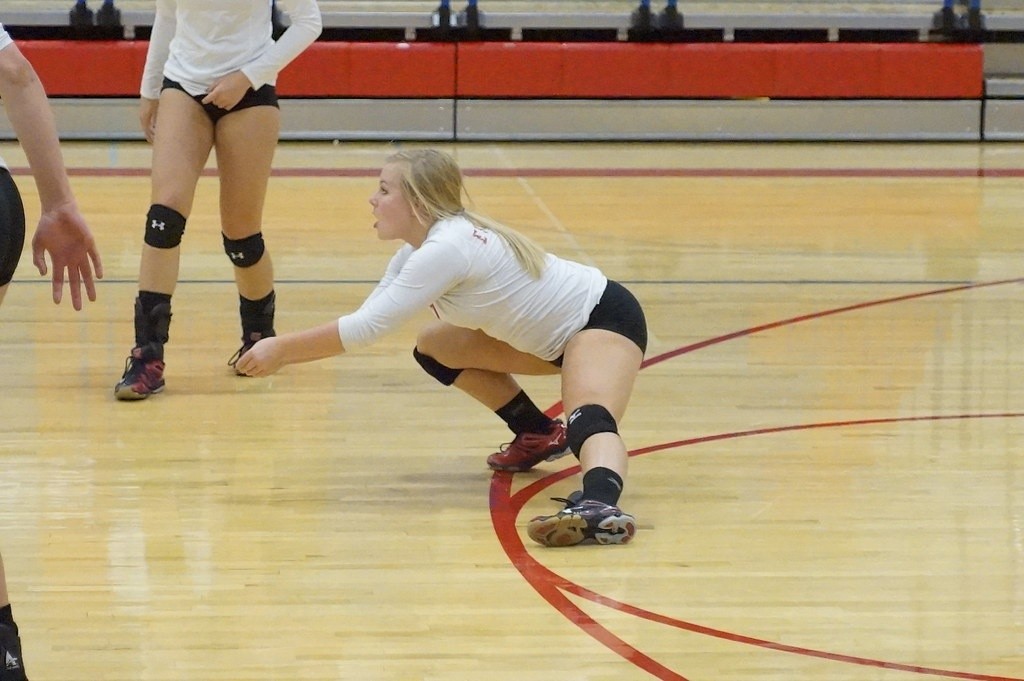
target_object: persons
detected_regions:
[238,149,647,549]
[0,16,105,681]
[114,1,322,402]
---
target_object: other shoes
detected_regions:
[0,622,28,681]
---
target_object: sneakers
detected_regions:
[114,348,165,399]
[228,329,276,376]
[487,418,572,471]
[527,498,636,547]
